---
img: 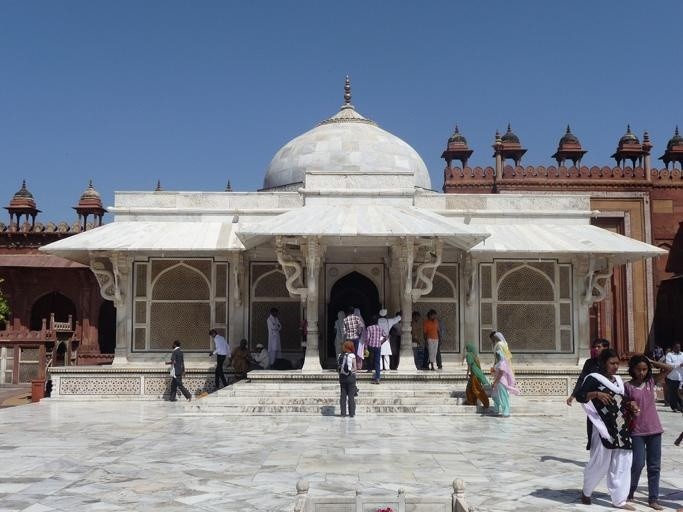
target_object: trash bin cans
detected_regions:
[31,379,46,403]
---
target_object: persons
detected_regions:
[266,307,282,364]
[332,306,445,370]
[363,315,388,384]
[252,343,269,369]
[337,340,359,418]
[163,340,192,402]
[460,332,519,418]
[298,318,308,358]
[564,337,682,511]
[227,339,251,372]
[207,328,231,387]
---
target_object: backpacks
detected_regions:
[336,353,350,376]
